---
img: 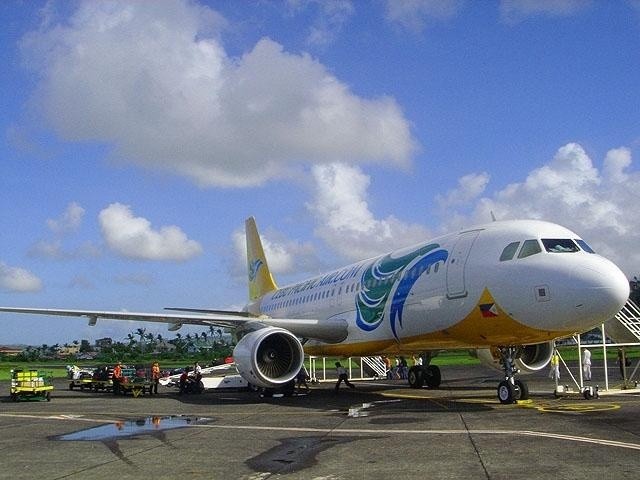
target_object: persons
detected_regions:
[551,352,560,381]
[582,347,591,381]
[615,346,627,380]
[383,355,423,380]
[153,417,160,429]
[116,421,124,431]
[112,353,234,396]
[297,367,312,395]
[334,362,356,392]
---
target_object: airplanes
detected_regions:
[0,213,632,407]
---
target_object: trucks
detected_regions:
[8,367,54,402]
